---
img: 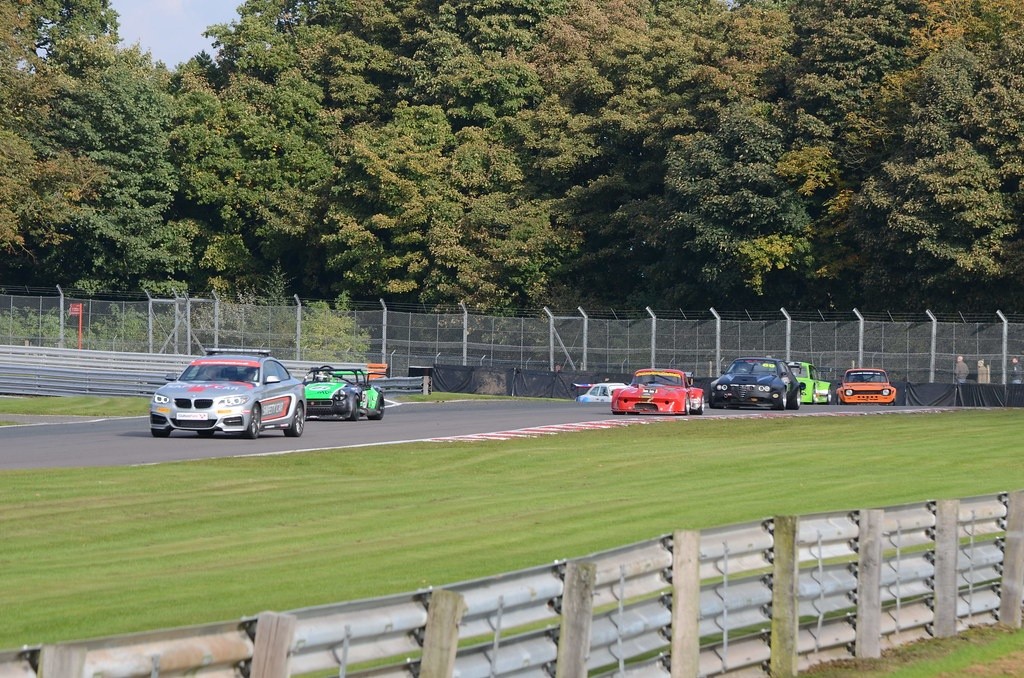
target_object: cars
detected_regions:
[761,361,831,404]
[835,369,897,406]
[708,356,806,410]
[574,382,627,401]
[300,366,387,421]
[147,348,308,439]
[609,367,705,416]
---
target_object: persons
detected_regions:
[1010,356,1022,384]
[977,360,987,384]
[316,371,330,382]
[955,356,969,383]
[244,368,258,382]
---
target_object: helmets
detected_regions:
[247,368,258,381]
